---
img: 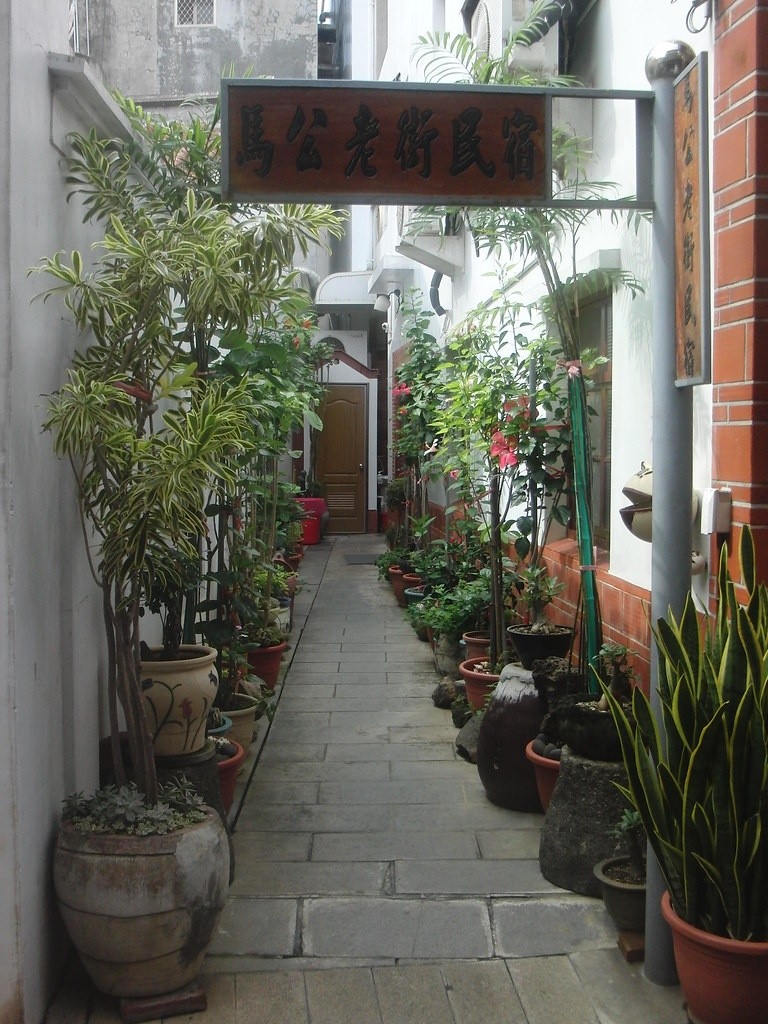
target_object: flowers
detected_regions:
[485,346,609,627]
[392,382,439,568]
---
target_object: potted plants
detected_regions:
[47,36,768,1024]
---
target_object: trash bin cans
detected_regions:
[294,498,324,544]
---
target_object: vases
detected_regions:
[505,624,574,678]
[388,566,409,608]
